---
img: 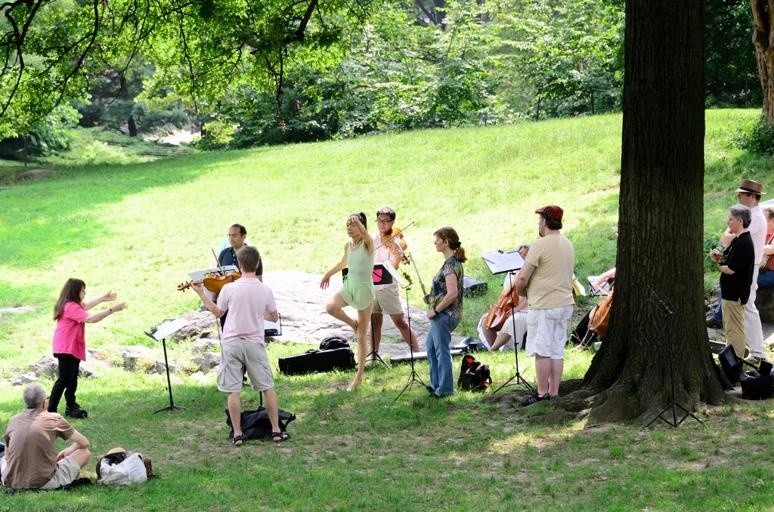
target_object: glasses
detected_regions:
[374,219,393,226]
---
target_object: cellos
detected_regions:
[588,286,615,335]
[484,277,519,331]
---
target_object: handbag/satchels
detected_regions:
[457,354,492,391]
[225,404,297,444]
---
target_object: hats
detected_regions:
[533,205,564,220]
[734,178,767,196]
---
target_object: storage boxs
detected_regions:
[279,346,357,378]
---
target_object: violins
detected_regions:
[177,272,242,292]
[380,228,410,265]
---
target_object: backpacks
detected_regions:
[321,335,351,350]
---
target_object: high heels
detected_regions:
[347,380,362,392]
[354,320,359,336]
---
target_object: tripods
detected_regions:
[152,339,185,414]
[485,279,538,401]
[392,315,434,404]
[355,320,390,373]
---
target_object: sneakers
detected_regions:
[519,390,549,408]
[742,354,770,363]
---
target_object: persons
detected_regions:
[707,178,774,360]
[47,278,126,419]
[320,212,375,390]
[369,207,420,359]
[0,382,91,490]
[426,226,464,397]
[516,206,574,405]
[190,247,289,446]
[217,223,262,380]
[478,244,530,352]
[586,266,617,334]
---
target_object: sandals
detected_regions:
[231,433,248,447]
[272,430,290,444]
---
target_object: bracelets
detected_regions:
[433,305,439,315]
[109,307,113,314]
